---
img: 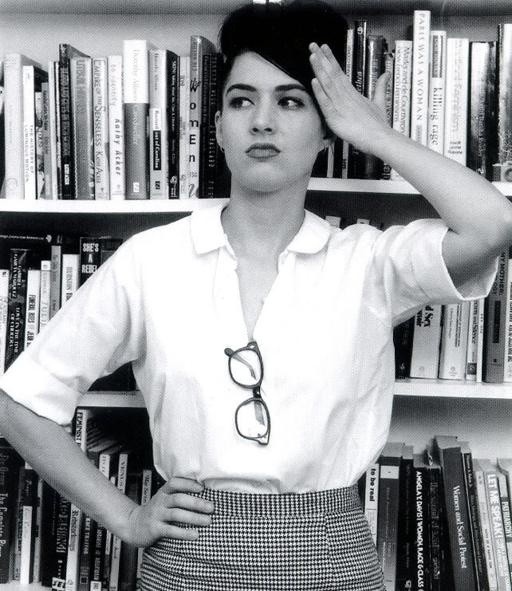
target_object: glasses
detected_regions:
[224,341,271,446]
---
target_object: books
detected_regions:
[358,433,512,591]
[0,32,224,201]
[325,8,512,183]
[393,245,512,384]
[0,408,164,591]
[0,232,136,394]
